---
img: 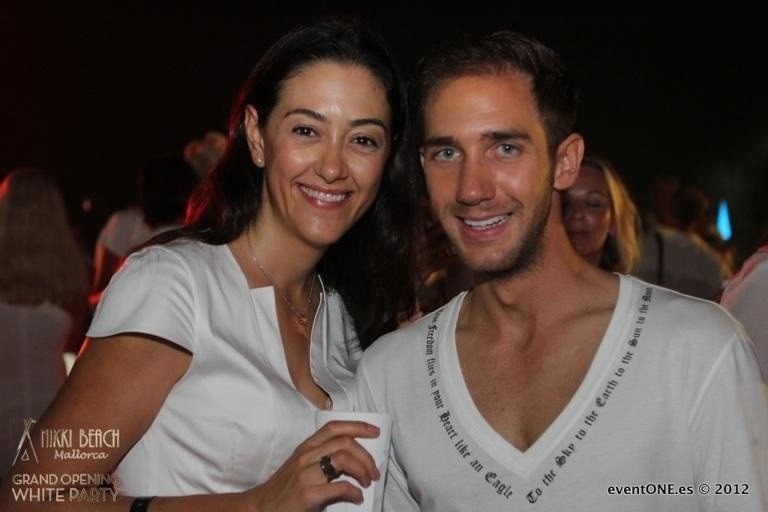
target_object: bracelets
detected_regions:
[130,496,154,511]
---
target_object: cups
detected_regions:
[316,410,394,512]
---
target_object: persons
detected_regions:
[1,166,87,483]
[88,119,765,350]
[0,26,410,512]
[352,30,767,511]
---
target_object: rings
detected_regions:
[320,453,338,481]
